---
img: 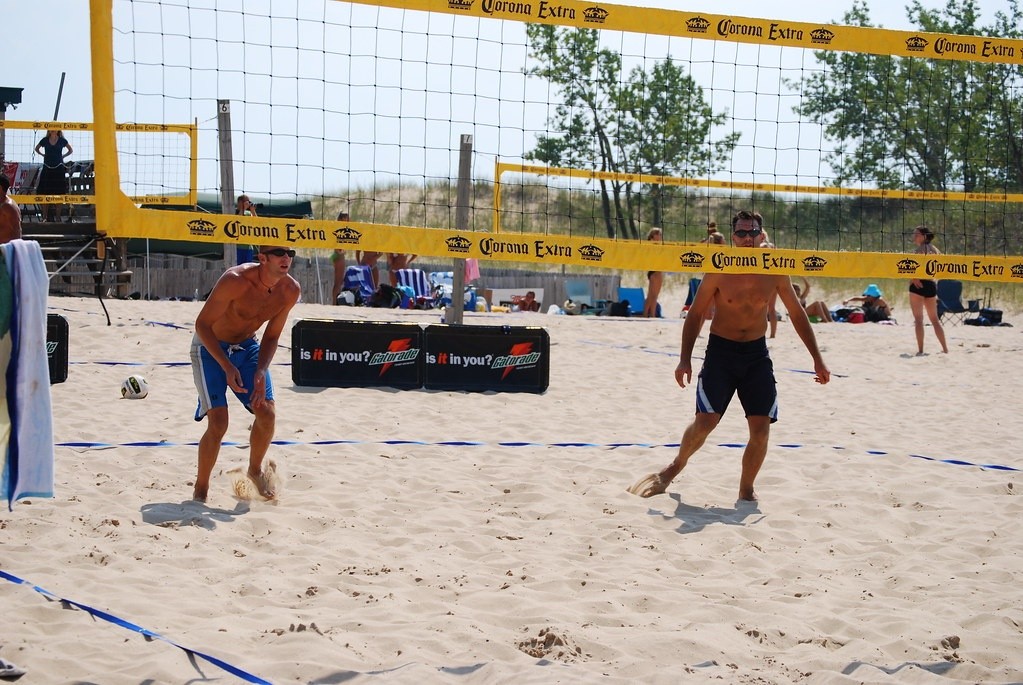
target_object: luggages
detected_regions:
[981,287,1003,325]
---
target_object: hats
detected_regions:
[862,285,881,298]
[564,299,576,310]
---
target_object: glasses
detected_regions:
[734,230,761,237]
[260,248,296,257]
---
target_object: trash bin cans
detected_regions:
[44,313,70,386]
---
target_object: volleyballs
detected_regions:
[121,375,149,400]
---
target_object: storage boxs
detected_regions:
[421,325,550,393]
[416,296,433,309]
[980,307,1004,323]
[477,289,492,303]
[486,304,491,312]
[848,312,864,323]
[336,297,352,305]
[291,320,421,387]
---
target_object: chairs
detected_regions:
[962,298,982,325]
[393,269,434,309]
[9,164,73,223]
[935,280,966,327]
[617,287,646,317]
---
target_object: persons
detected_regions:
[518,291,632,317]
[234,194,260,265]
[330,212,418,307]
[189,245,302,503]
[908,225,949,355]
[679,221,892,339]
[35,130,73,223]
[640,210,831,501]
[0,171,23,244]
[642,227,663,318]
